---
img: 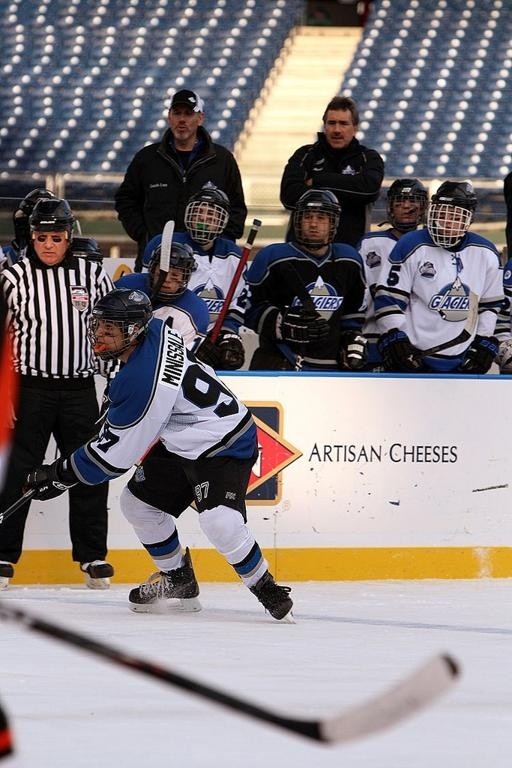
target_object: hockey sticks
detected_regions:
[1,602,458,744]
[413,289,479,361]
[148,220,175,299]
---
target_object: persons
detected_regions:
[113,231,245,370]
[22,286,294,620]
[1,198,123,579]
[353,178,429,372]
[0,187,57,272]
[214,189,374,371]
[115,89,248,271]
[279,96,385,248]
[141,188,251,335]
[0,300,16,754]
[494,257,511,374]
[373,180,505,375]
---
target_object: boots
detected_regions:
[130,545,199,604]
[248,569,292,620]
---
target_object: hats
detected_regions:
[169,89,202,112]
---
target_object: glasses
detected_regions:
[37,235,62,243]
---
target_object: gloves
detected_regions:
[192,329,245,370]
[23,456,80,501]
[456,334,499,374]
[342,330,370,369]
[274,306,331,346]
[376,328,420,374]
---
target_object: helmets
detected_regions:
[15,188,75,231]
[293,188,341,249]
[426,181,478,248]
[70,236,103,261]
[88,288,154,360]
[184,188,230,247]
[387,179,428,234]
[149,242,196,301]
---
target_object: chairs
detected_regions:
[315,3,511,180]
[3,1,307,186]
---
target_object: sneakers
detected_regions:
[1,564,13,577]
[79,561,114,579]
